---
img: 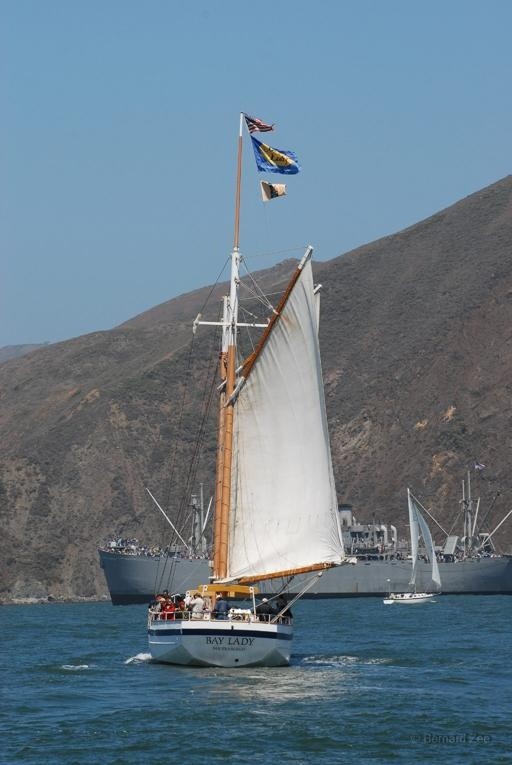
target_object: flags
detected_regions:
[259,179,288,202]
[248,134,302,175]
[473,458,487,471]
[242,111,275,134]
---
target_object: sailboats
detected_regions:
[146,109,359,669]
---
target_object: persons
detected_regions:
[100,534,215,562]
[257,598,275,623]
[276,595,293,623]
[152,590,232,621]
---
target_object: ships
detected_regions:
[98,455,512,607]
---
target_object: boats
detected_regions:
[382,591,437,605]
